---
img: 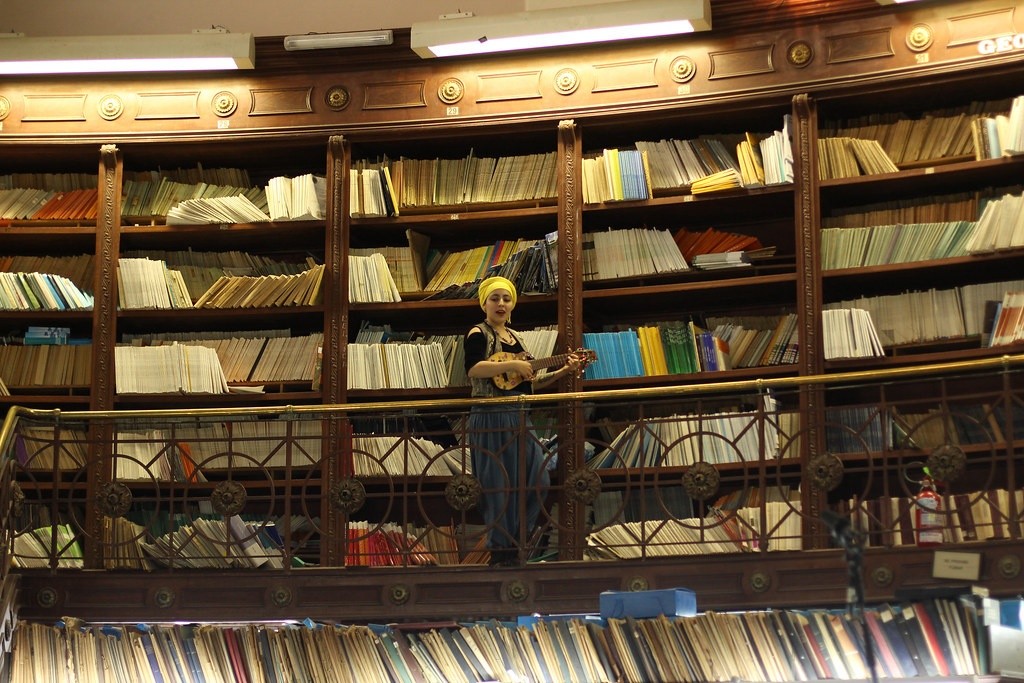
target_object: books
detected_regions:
[0,172,98,569]
[345,148,558,567]
[582,114,802,561]
[9,597,989,683]
[102,161,326,571]
[815,95,1024,548]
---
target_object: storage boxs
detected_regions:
[599,588,698,618]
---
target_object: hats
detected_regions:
[478,276,517,313]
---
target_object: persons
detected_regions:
[463,276,579,569]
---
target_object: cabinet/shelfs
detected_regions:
[0,0,1024,562]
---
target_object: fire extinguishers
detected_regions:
[915,466,945,545]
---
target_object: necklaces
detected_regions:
[496,327,513,344]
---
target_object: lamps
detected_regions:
[0,25,256,74]
[411,0,712,60]
[284,30,393,51]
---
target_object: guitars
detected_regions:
[486,348,598,392]
